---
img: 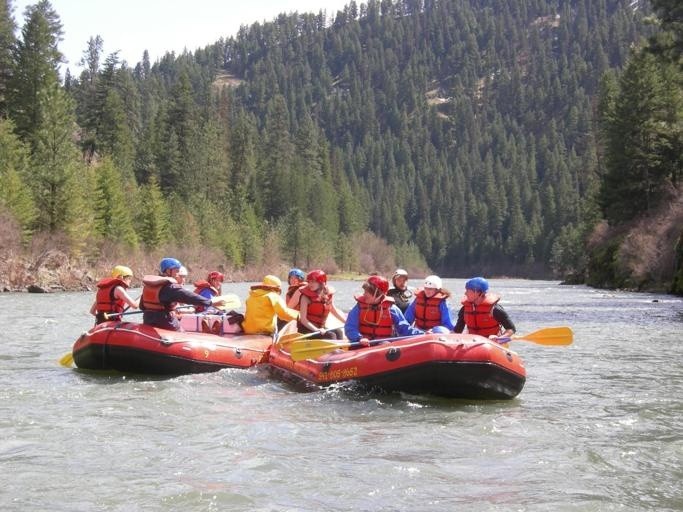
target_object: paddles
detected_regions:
[60,293,141,368]
[278,324,346,350]
[288,335,419,362]
[494,328,573,346]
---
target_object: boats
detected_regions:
[265,331,528,404]
[69,318,272,375]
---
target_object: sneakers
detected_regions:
[201,318,220,335]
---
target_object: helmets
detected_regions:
[111,258,327,289]
[366,268,489,294]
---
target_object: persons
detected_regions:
[404,276,454,331]
[449,276,516,348]
[277,268,306,333]
[385,269,413,313]
[89,265,144,326]
[344,274,434,348]
[240,274,300,335]
[295,268,348,340]
[192,270,225,313]
[176,265,188,308]
[138,256,225,333]
